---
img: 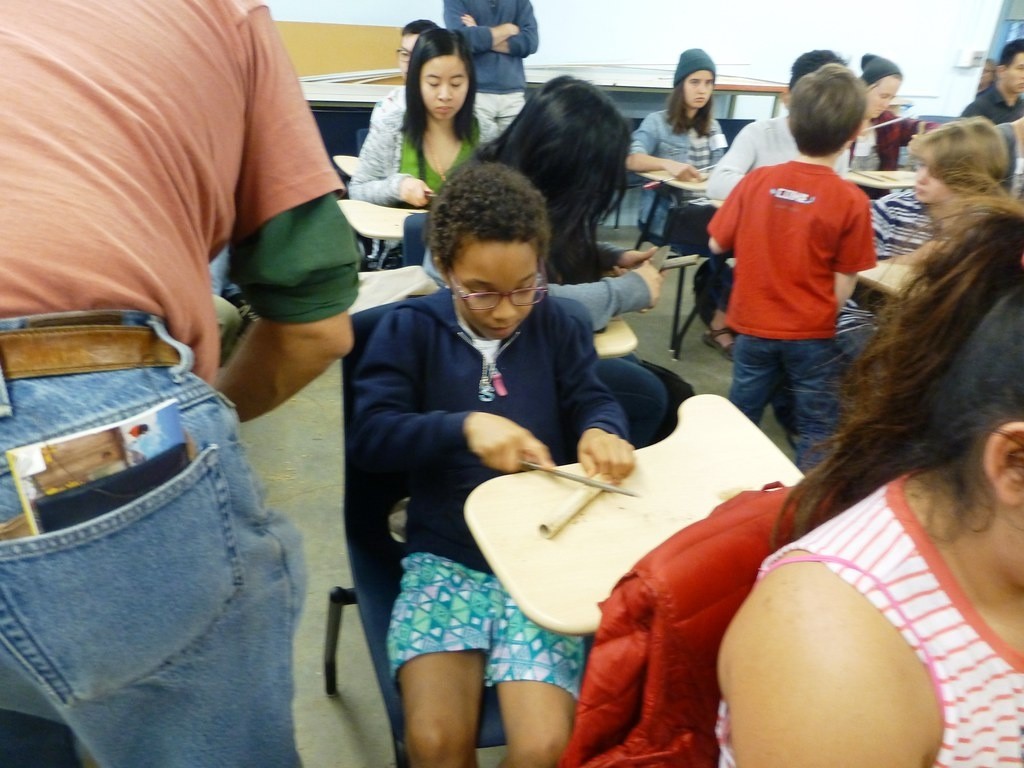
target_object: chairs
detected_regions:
[636,177,712,352]
[404,211,429,266]
[635,171,709,193]
[325,298,595,768]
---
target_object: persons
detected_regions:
[959,38,1024,138]
[367,18,439,129]
[439,1,540,150]
[419,76,667,336]
[0,0,361,768]
[869,116,1016,255]
[704,64,878,472]
[994,117,1023,201]
[352,160,639,768]
[846,51,941,170]
[715,186,1024,768]
[624,46,740,364]
[703,46,851,203]
[977,58,997,94]
[346,24,500,208]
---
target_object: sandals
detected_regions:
[704,326,736,362]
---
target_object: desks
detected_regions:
[844,170,920,190]
[338,200,428,241]
[855,261,912,299]
[465,393,805,636]
[332,155,359,175]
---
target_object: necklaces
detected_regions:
[426,131,446,182]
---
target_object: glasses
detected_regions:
[448,256,548,311]
[397,49,411,62]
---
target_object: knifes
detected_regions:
[638,246,671,273]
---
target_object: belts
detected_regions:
[0,326,179,379]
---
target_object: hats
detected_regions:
[674,48,716,87]
[860,54,903,86]
